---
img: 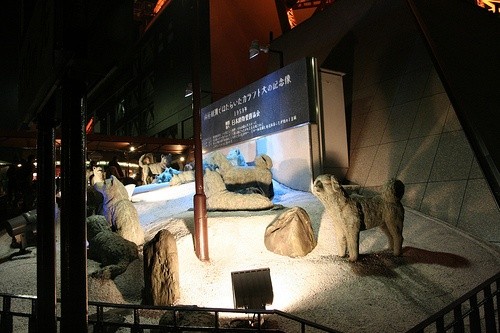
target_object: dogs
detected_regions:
[139,151,173,184]
[200,152,278,212]
[307,172,408,263]
[91,166,145,246]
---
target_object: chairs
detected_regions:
[6,209,36,259]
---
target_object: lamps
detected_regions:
[231,268,273,326]
[131,0,158,24]
